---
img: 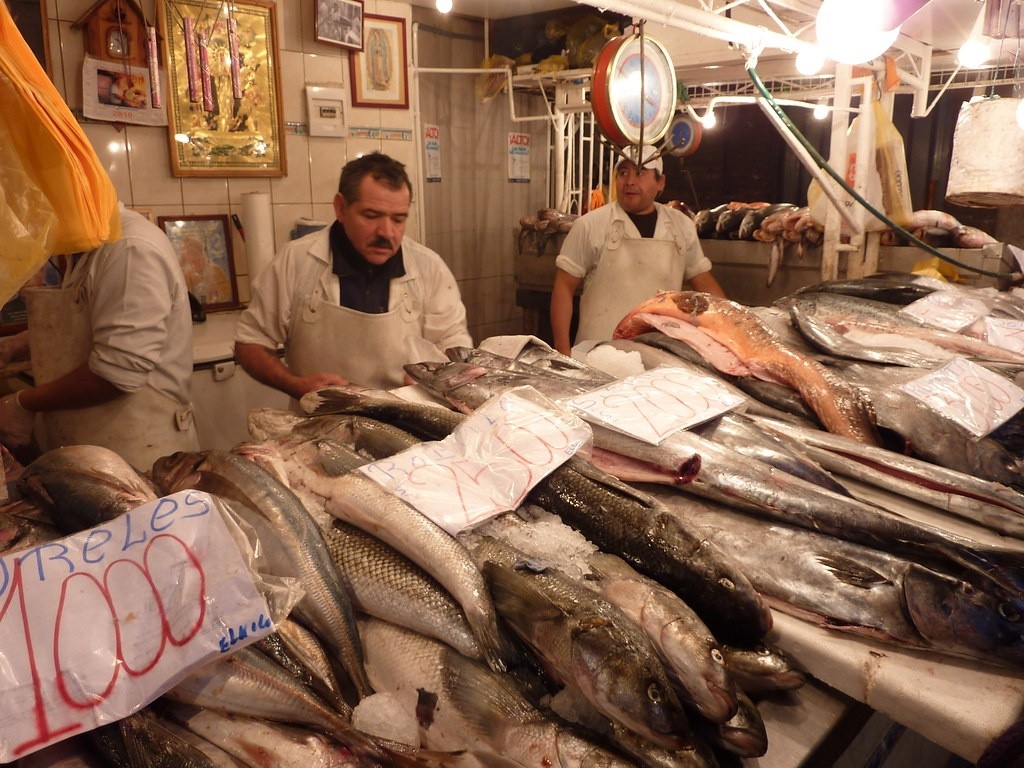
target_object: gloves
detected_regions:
[0,390,38,449]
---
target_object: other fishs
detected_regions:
[663,199,825,290]
[0,272,1024,768]
[880,210,999,249]
[517,207,581,258]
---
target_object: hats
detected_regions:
[614,145,663,176]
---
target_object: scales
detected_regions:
[667,114,702,155]
[592,33,677,175]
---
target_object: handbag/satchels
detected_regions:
[805,101,915,237]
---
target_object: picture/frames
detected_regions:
[128,208,153,224]
[155,0,289,179]
[348,13,410,109]
[157,214,240,311]
[313,0,364,52]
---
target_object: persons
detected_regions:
[550,145,728,358]
[0,197,202,478]
[320,1,361,43]
[232,150,474,410]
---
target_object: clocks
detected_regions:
[67,0,155,132]
